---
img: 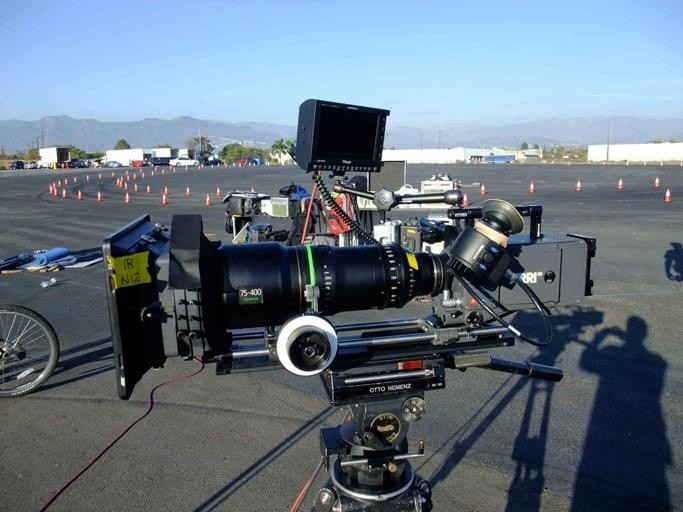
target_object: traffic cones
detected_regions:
[459,173,678,208]
[48,167,222,206]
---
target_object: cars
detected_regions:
[6,159,123,170]
[133,155,265,166]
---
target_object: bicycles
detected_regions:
[0,304,60,400]
